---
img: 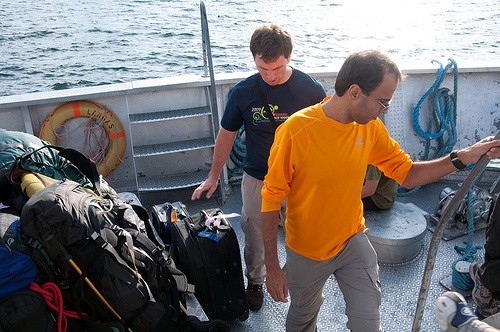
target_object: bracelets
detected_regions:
[450,150,468,170]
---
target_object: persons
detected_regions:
[436,192,500,332]
[192,23,327,311]
[361,164,398,211]
[261,51,500,332]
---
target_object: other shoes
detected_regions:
[435,291,479,332]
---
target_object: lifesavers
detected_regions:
[39,100,127,179]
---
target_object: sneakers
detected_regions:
[469,262,492,307]
[246,283,264,311]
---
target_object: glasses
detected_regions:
[349,83,394,113]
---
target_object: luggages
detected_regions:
[188,207,249,322]
[152,200,197,263]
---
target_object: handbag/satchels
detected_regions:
[0,128,209,332]
